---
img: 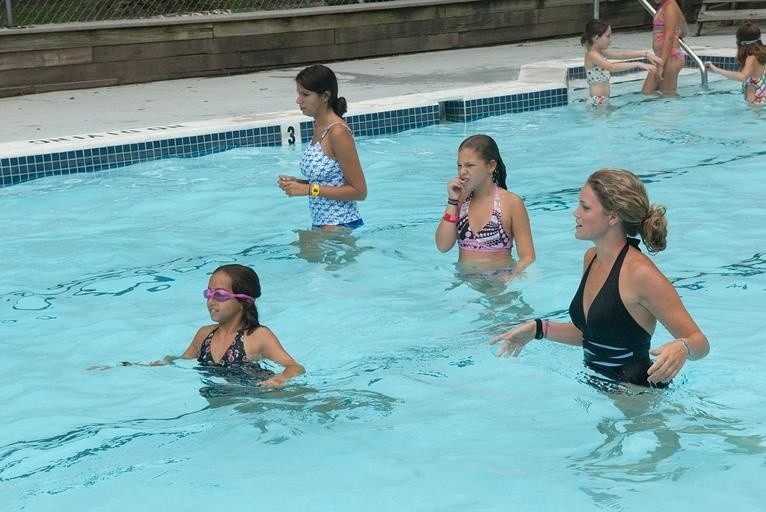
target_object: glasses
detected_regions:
[203,288,256,303]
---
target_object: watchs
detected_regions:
[310,183,320,197]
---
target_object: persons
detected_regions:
[640,0,688,97]
[704,24,766,104]
[490,167,711,394]
[278,63,368,231]
[86,264,306,397]
[581,19,665,112]
[436,135,535,282]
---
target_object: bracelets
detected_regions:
[535,319,547,339]
[442,212,459,224]
[645,51,649,58]
[677,337,692,357]
[447,199,459,206]
[308,184,311,195]
